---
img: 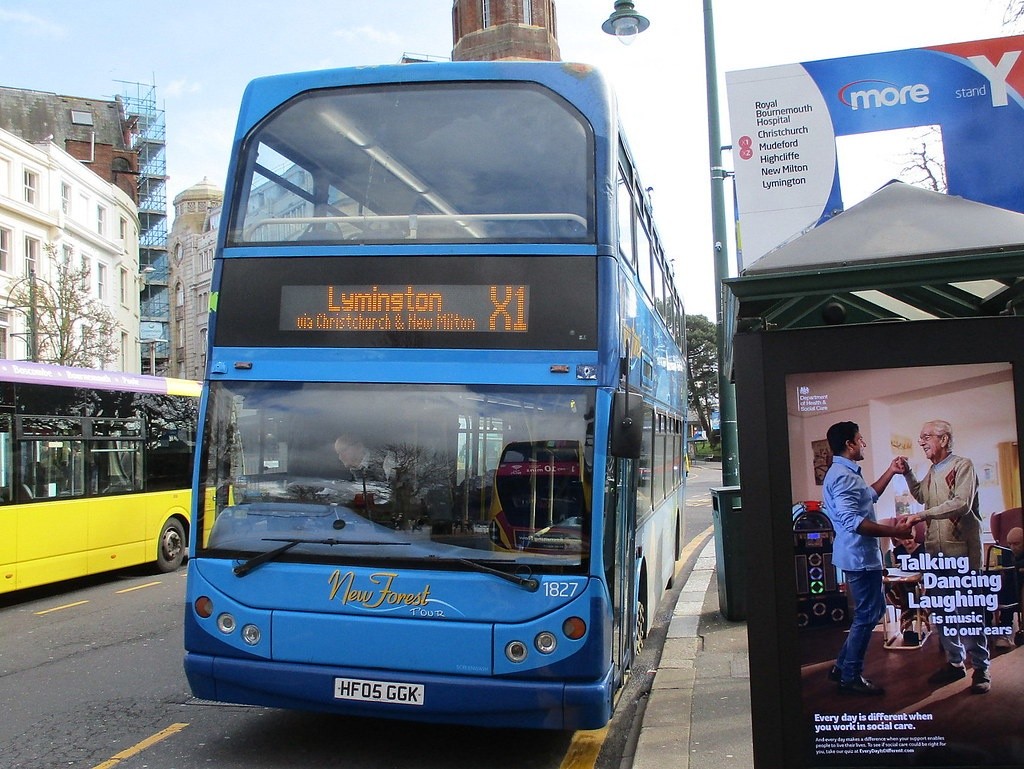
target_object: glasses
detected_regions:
[918,435,942,442]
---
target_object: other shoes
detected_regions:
[928,664,966,684]
[996,637,1012,647]
[972,669,991,694]
[904,630,926,645]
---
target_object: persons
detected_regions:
[820,420,990,700]
[333,430,419,523]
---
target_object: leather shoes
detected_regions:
[828,669,885,698]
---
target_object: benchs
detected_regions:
[703,454,715,460]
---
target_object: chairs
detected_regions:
[456,469,495,514]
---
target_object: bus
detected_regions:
[0,361,202,594]
[185,61,690,729]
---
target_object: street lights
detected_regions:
[601,0,737,485]
